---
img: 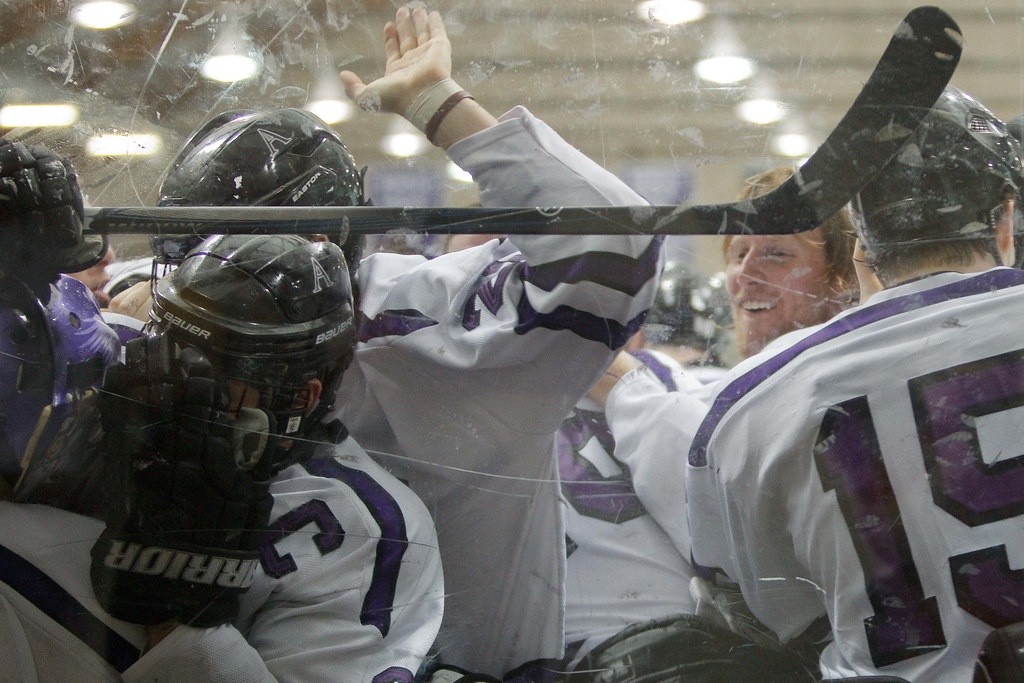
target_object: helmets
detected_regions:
[147,234,354,438]
[158,106,370,263]
[0,274,122,502]
[853,83,1022,251]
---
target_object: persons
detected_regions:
[0,1,667,683]
[559,85,1023,683]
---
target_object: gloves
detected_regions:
[92,333,273,628]
[1,137,85,296]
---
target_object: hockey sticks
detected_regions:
[70,3,967,239]
